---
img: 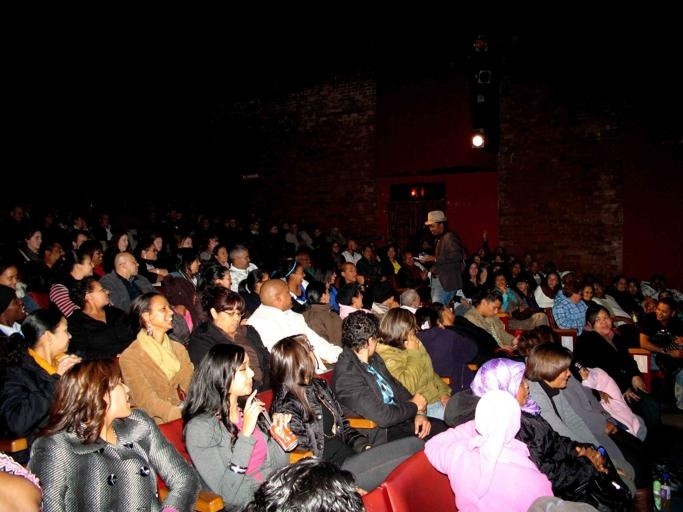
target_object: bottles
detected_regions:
[661,472,671,512]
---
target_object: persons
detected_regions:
[1,201,683,512]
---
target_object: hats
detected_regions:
[560,270,575,280]
[0,284,16,313]
[425,211,446,225]
